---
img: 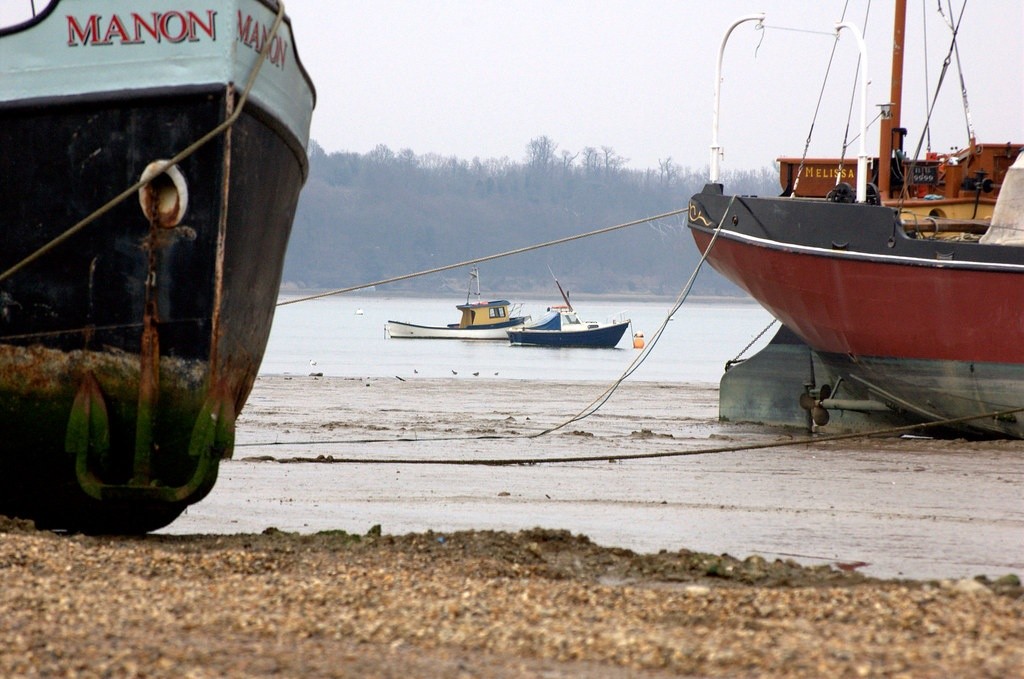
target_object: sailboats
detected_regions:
[685,0,1023,440]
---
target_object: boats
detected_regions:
[384,262,531,341]
[505,264,631,348]
[1,0,316,533]
